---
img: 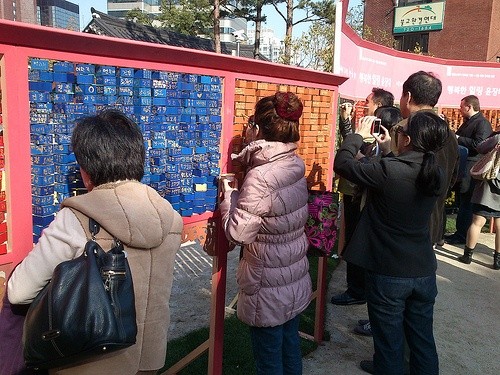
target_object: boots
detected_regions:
[493,250,500,270]
[458,246,475,265]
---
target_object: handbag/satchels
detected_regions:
[304,190,340,259]
[20,217,138,371]
[469,141,500,181]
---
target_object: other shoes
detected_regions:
[443,231,467,244]
[353,319,373,336]
[360,360,377,374]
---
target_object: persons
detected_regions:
[216,92,312,375]
[444,95,500,270]
[331,72,459,375]
[6,109,183,375]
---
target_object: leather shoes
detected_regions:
[331,288,367,305]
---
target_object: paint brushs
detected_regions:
[340,105,369,108]
[241,113,250,119]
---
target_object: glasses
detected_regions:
[394,125,407,137]
[248,115,257,126]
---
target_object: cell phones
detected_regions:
[371,118,382,135]
